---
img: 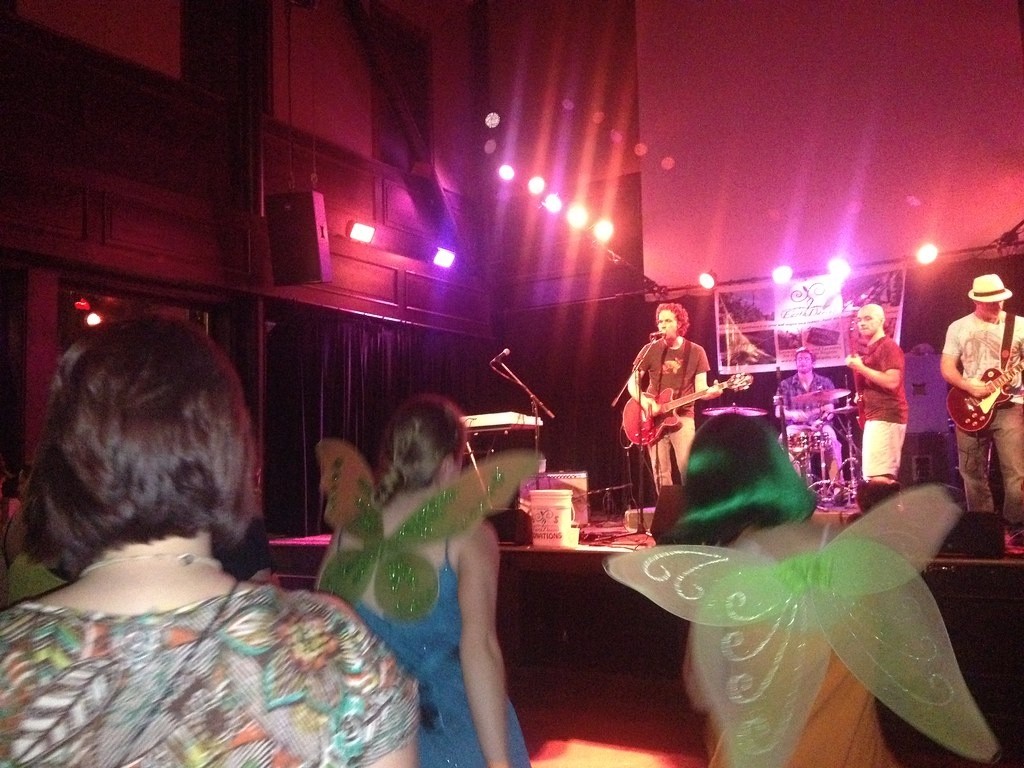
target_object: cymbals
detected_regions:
[830,405,856,414]
[701,402,768,417]
[790,386,851,402]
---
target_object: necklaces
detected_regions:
[77,551,223,580]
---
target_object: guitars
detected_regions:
[848,309,866,431]
[945,358,1024,434]
[622,371,754,446]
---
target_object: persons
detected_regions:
[1,315,419,768]
[672,412,900,768]
[0,464,277,606]
[845,303,910,484]
[939,274,1024,526]
[775,347,842,494]
[627,303,723,497]
[316,394,531,768]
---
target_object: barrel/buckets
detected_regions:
[529,489,576,546]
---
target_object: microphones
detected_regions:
[649,329,667,338]
[490,348,510,365]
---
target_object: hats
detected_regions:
[968,274,1013,302]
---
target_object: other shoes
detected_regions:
[1005,519,1024,546]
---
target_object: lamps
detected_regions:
[698,269,717,288]
[344,219,377,243]
[429,245,458,270]
[772,260,794,282]
[478,103,503,129]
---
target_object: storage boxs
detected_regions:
[518,470,590,526]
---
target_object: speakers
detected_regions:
[485,509,531,544]
[939,510,1006,559]
[265,190,335,286]
[650,485,687,545]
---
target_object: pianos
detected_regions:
[458,412,545,476]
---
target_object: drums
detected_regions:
[787,432,830,452]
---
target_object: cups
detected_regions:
[537,459,546,473]
